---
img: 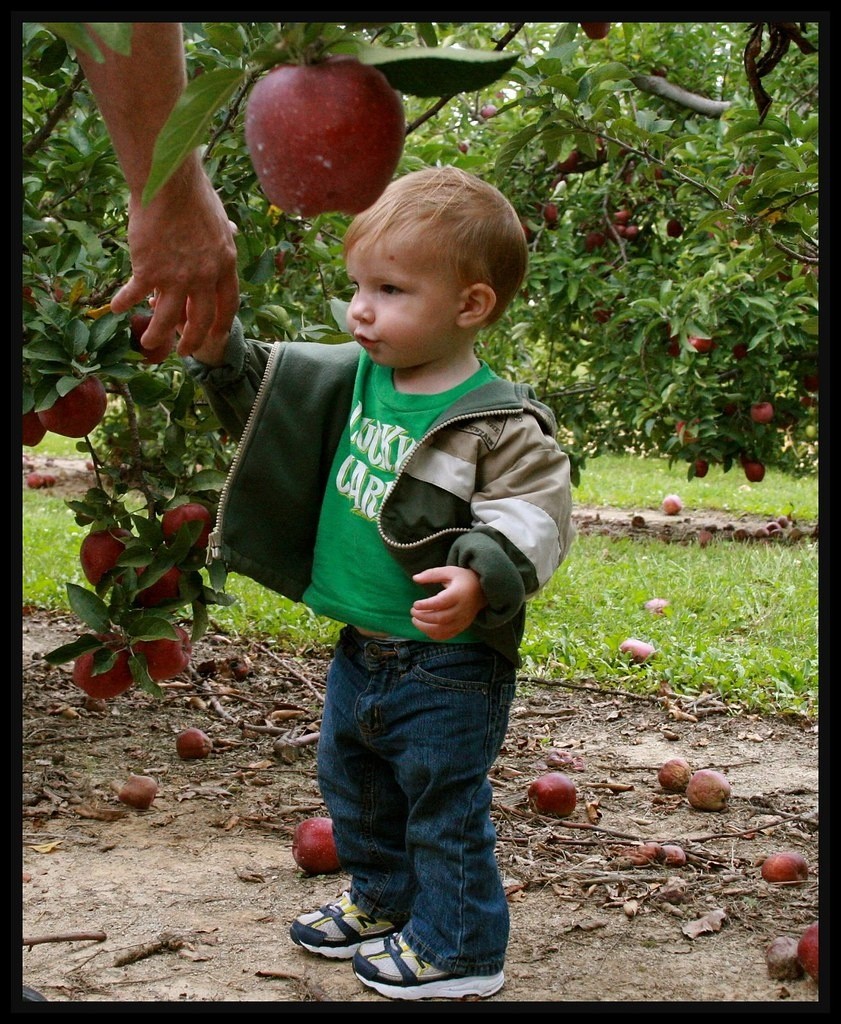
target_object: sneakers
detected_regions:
[352,932,504,1000]
[290,891,403,959]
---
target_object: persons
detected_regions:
[149,165,572,1001]
[62,23,238,366]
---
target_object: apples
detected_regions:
[633,495,789,545]
[761,853,808,885]
[118,775,157,810]
[797,923,818,985]
[80,528,135,587]
[92,632,126,643]
[163,502,210,548]
[644,599,670,613]
[73,644,134,700]
[133,627,192,679]
[765,936,805,979]
[136,566,180,607]
[685,771,732,810]
[528,772,577,818]
[621,640,653,662]
[658,758,692,792]
[84,697,105,711]
[285,817,340,870]
[21,22,819,483]
[176,728,212,758]
